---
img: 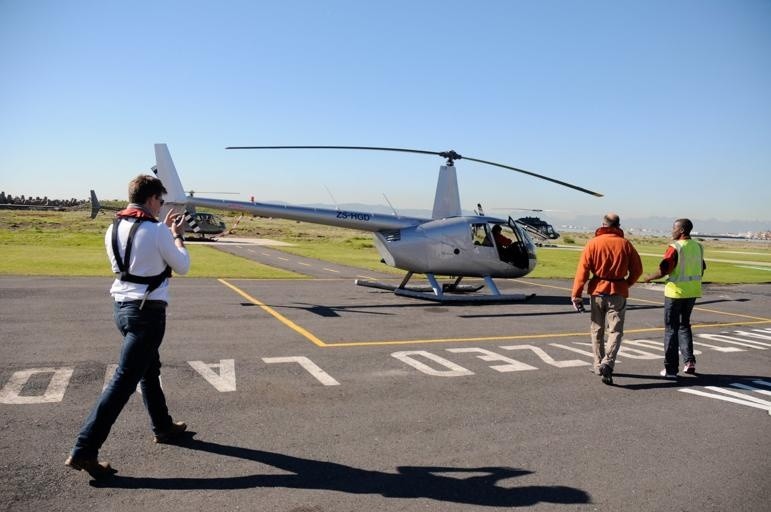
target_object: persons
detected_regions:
[63,172,191,481]
[643,217,708,378]
[569,211,643,386]
[480,224,514,260]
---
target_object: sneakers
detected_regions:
[64,454,111,481]
[660,368,678,378]
[683,360,696,373]
[589,363,614,385]
[153,421,188,444]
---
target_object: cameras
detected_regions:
[576,301,585,312]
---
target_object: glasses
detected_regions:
[156,197,165,208]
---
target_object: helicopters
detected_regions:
[91,146,605,303]
[487,206,569,248]
[89,186,240,243]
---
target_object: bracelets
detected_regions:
[172,233,186,241]
